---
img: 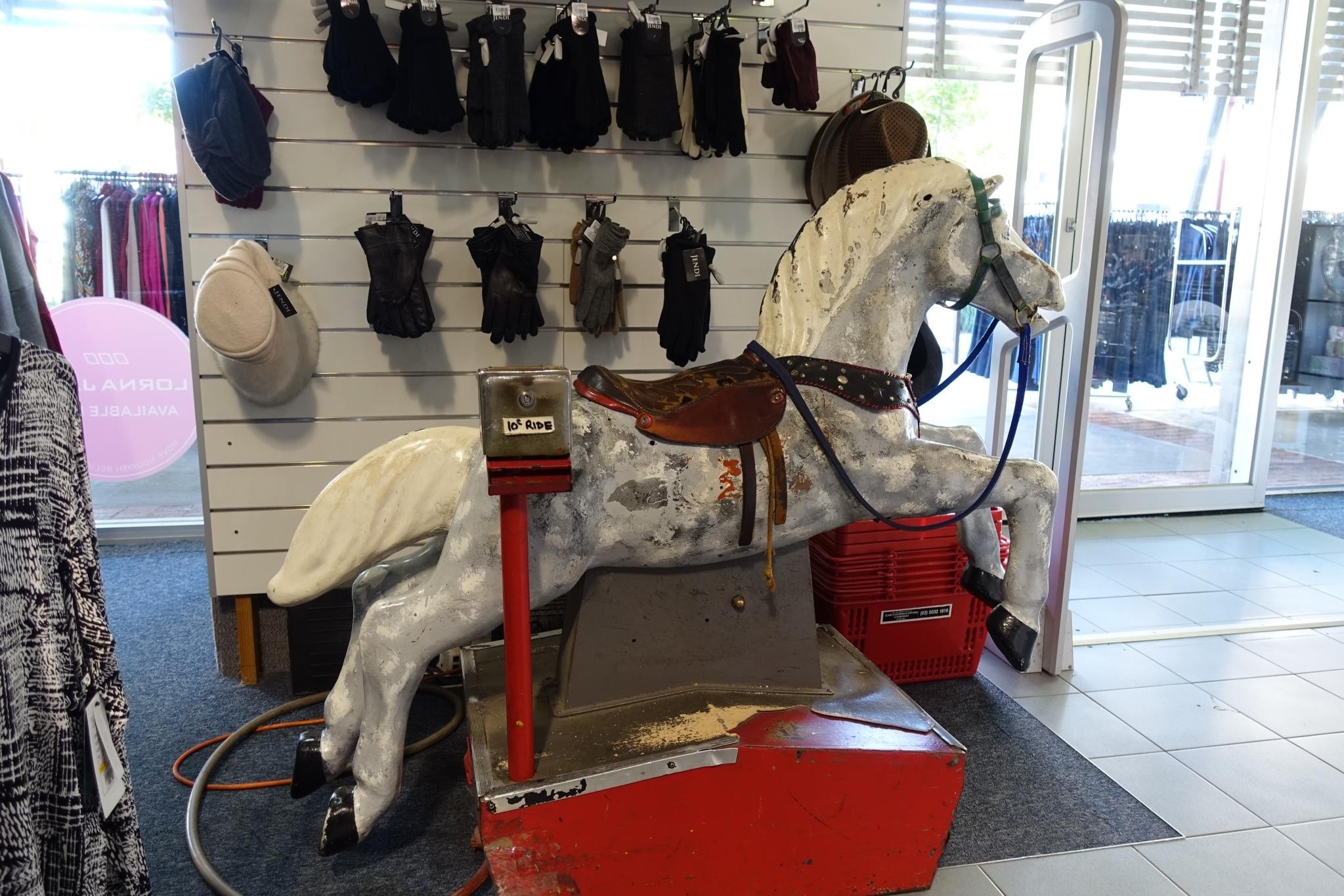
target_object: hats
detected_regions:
[806,89,930,212]
[193,239,321,406]
[173,56,272,202]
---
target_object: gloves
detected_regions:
[319,0,821,367]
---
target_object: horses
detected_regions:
[266,156,1066,853]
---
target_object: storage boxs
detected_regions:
[809,512,1009,682]
